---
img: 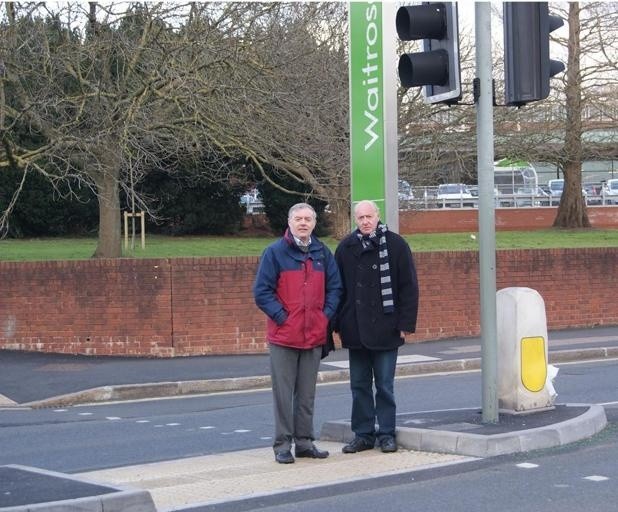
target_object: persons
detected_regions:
[254,203,344,464]
[333,201,419,454]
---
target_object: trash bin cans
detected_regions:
[494,286,556,416]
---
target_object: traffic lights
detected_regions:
[504,1,566,108]
[394,2,463,107]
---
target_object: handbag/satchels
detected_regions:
[321,322,335,359]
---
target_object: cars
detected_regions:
[239,187,266,215]
[323,178,618,215]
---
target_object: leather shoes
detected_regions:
[275,450,294,464]
[342,437,374,452]
[380,437,396,452]
[296,445,329,458]
